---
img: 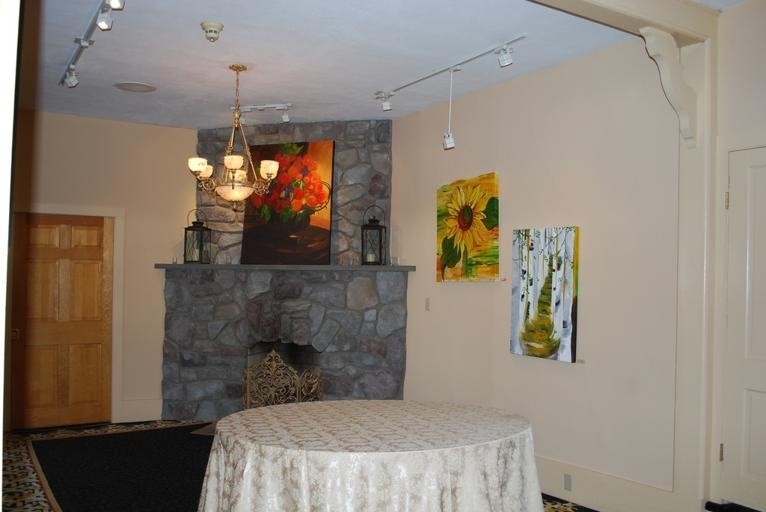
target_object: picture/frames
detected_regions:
[436,172,501,285]
[240,139,335,265]
[509,226,579,363]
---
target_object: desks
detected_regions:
[198,400,543,512]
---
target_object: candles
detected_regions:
[366,253,376,261]
[191,249,201,262]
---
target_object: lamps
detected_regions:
[105,0,126,11]
[63,65,80,88]
[381,97,392,111]
[188,63,280,212]
[239,117,252,125]
[442,68,464,149]
[281,108,291,122]
[97,7,112,32]
[494,46,515,67]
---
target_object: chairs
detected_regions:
[299,368,323,401]
[241,349,300,410]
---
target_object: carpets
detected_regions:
[2,418,594,512]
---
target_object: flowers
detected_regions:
[249,143,327,225]
